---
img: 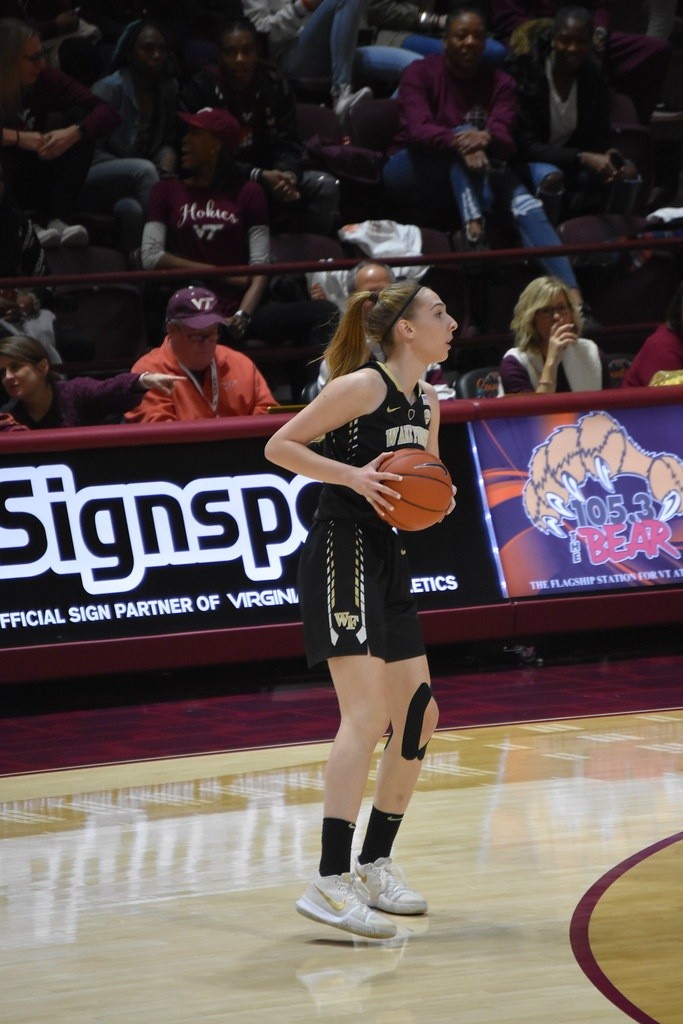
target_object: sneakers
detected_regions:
[33,223,60,249]
[295,869,397,939]
[351,855,427,914]
[334,85,373,130]
[47,219,89,249]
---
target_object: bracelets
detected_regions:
[138,372,154,389]
[576,150,582,167]
[421,11,431,33]
[429,14,437,33]
[14,128,20,146]
[595,27,607,38]
[436,14,442,30]
[538,379,557,386]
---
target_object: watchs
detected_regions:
[234,309,251,325]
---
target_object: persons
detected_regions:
[266,282,458,940]
[0,0,683,432]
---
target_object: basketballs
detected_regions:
[374,447,453,532]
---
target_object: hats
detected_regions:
[166,286,229,330]
[177,107,240,149]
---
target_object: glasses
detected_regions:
[21,52,42,64]
[185,333,221,343]
[534,304,570,318]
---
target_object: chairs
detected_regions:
[0,36,683,411]
